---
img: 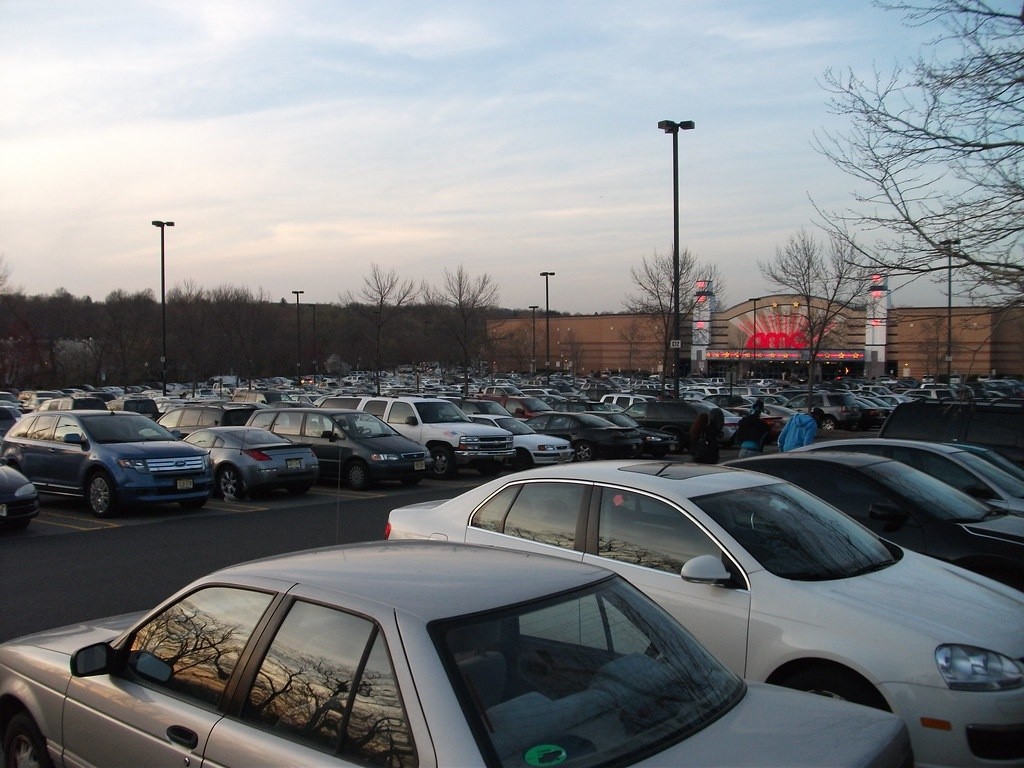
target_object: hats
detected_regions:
[811,408,825,420]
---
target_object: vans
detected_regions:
[244,408,430,492]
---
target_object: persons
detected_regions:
[729,400,772,458]
[777,408,826,452]
[687,412,719,463]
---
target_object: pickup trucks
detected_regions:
[318,392,517,480]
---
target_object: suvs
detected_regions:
[876,398,1024,450]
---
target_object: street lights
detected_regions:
[292,290,304,386]
[152,220,175,397]
[750,298,761,378]
[940,238,960,395]
[540,272,555,385]
[658,120,695,398]
[529,305,539,375]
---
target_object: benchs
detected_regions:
[480,648,679,761]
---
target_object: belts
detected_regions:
[740,447,759,451]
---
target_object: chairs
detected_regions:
[338,418,350,434]
[456,651,507,714]
[307,420,320,436]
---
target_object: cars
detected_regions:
[385,457,1024,768]
[1,538,914,768]
[0,360,1024,463]
[464,415,575,473]
[183,425,319,502]
[524,412,643,461]
[785,437,1024,511]
[720,449,1024,594]
[0,462,39,533]
[0,409,214,519]
